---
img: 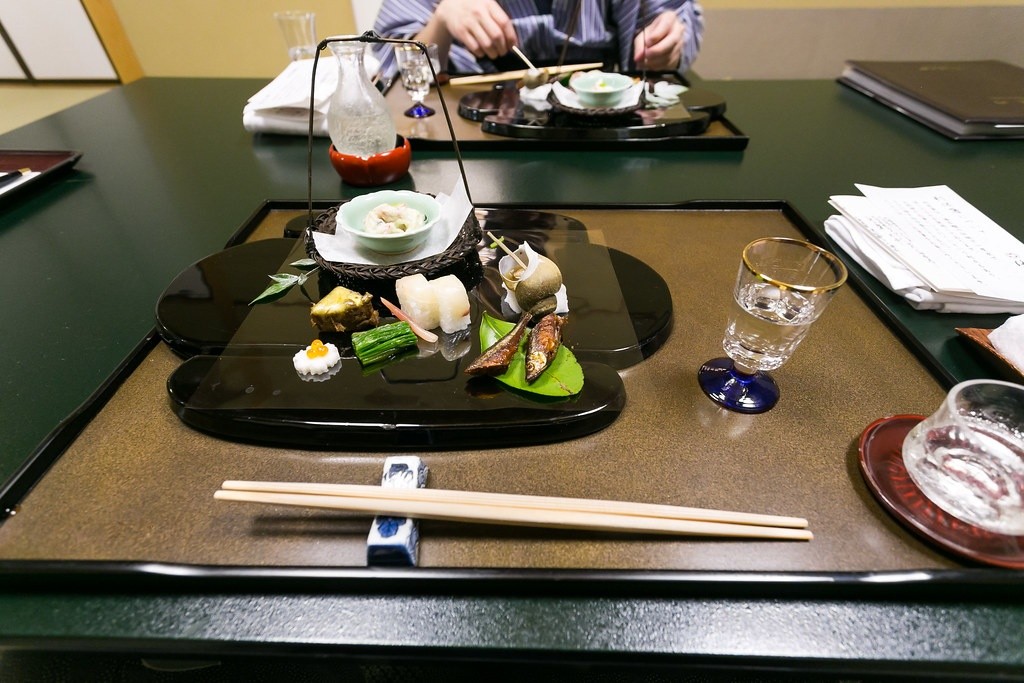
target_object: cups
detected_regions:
[271,13,318,61]
[902,380,1024,536]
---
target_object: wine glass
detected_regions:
[696,236,848,414]
[398,42,440,119]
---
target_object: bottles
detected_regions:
[328,36,395,156]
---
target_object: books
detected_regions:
[840,61,1024,142]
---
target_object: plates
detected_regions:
[858,413,1024,568]
[546,86,645,120]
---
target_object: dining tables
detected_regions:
[0,75,1024,683]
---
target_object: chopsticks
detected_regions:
[215,480,817,542]
[448,63,605,85]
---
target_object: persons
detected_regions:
[372,0,703,84]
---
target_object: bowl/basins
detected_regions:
[329,135,411,188]
[571,72,635,109]
[336,191,440,255]
